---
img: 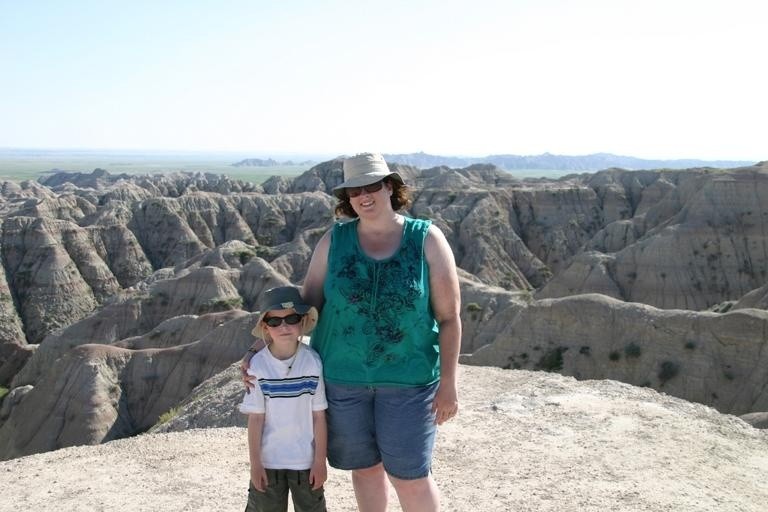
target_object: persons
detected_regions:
[240,150,462,512]
[238,284,329,512]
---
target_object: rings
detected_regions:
[242,376,245,382]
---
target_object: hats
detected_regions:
[252,287,319,341]
[333,152,406,199]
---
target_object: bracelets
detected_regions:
[246,347,257,353]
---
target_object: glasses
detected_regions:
[264,313,306,327]
[345,181,383,197]
